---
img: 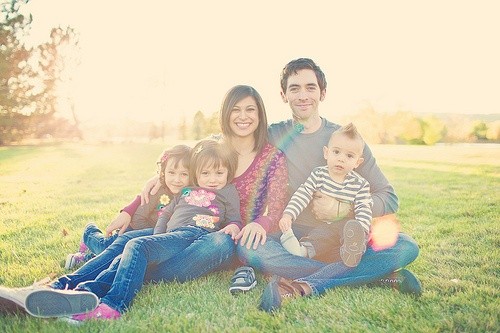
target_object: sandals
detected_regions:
[229,267,258,294]
[340,220,366,267]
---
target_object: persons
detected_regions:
[0,85,288,317]
[141,58,423,313]
[279,121,374,267]
[65,145,192,270]
[25,140,243,325]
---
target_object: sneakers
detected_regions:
[257,274,305,312]
[66,253,86,270]
[367,268,422,296]
[63,301,120,324]
[80,222,96,253]
[0,284,54,309]
[24,289,99,318]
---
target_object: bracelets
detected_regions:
[337,202,341,220]
